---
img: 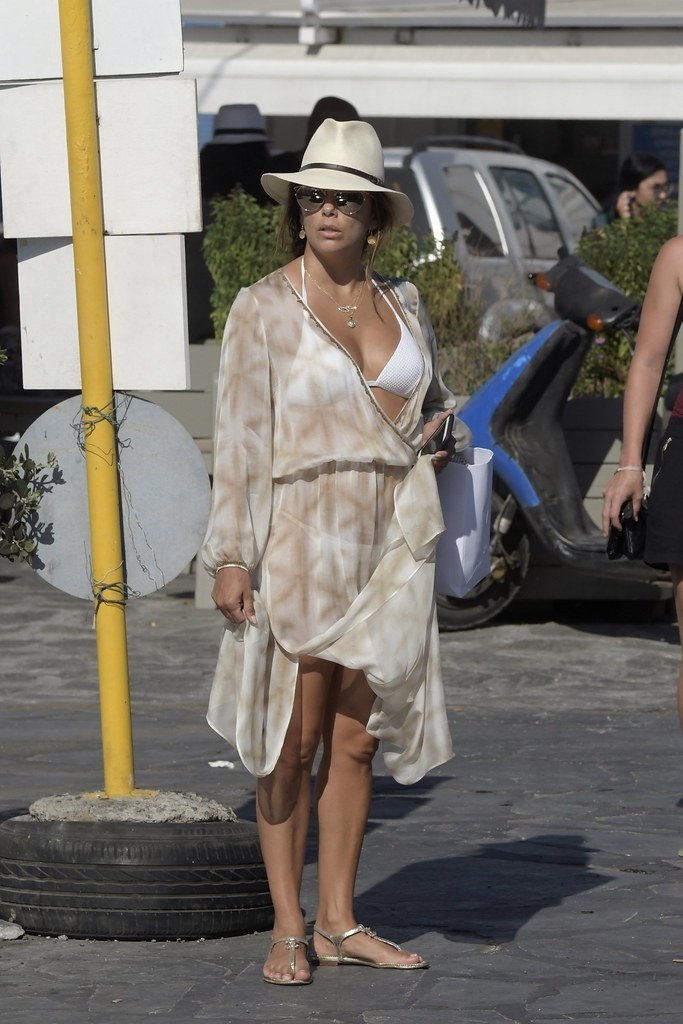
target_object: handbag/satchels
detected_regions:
[433,447,495,598]
[605,498,643,559]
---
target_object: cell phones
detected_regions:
[418,413,455,460]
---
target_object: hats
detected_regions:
[260,118,413,228]
[207,105,272,143]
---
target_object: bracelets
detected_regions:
[613,466,644,475]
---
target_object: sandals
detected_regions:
[307,923,428,969]
[261,935,310,986]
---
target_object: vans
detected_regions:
[376,136,612,353]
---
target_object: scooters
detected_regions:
[434,247,674,634]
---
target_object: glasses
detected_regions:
[292,184,374,214]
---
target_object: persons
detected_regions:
[603,231,683,860]
[195,117,458,984]
[594,152,670,226]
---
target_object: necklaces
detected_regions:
[306,270,367,328]
